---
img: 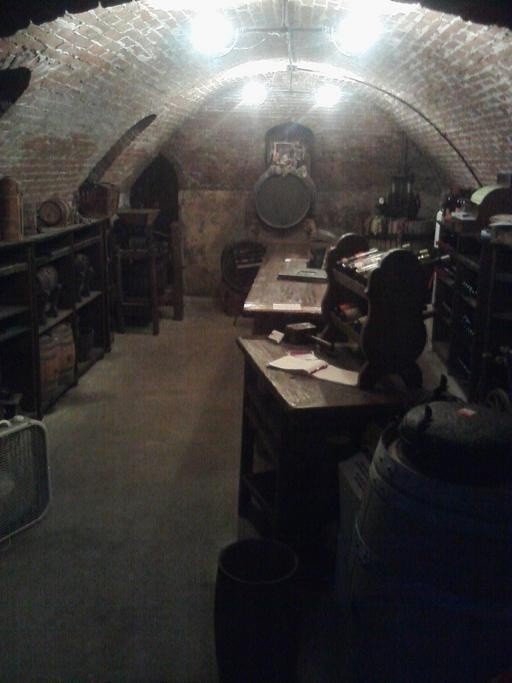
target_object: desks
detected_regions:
[242,241,334,318]
[241,331,429,506]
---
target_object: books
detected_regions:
[267,352,363,387]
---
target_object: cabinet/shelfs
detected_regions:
[322,235,426,390]
[431,218,512,405]
[1,219,114,421]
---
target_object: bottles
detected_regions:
[335,299,441,334]
[334,240,451,284]
[384,175,416,236]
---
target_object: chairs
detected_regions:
[221,240,267,326]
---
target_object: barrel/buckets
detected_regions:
[15,323,76,408]
[343,423,511,683]
